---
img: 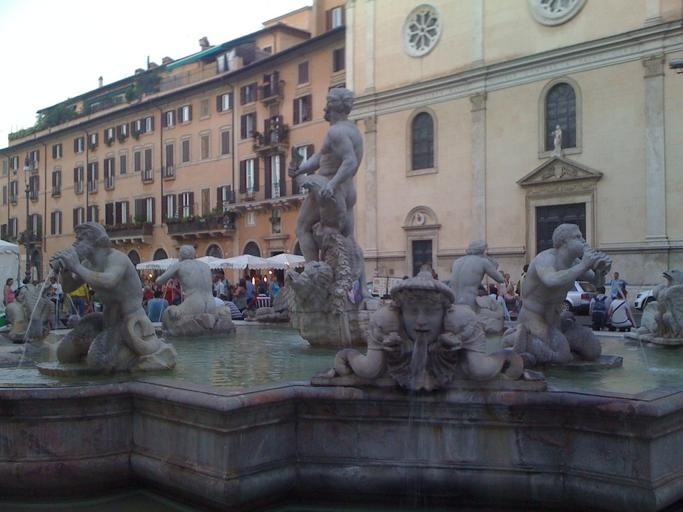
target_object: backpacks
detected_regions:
[592,296,607,322]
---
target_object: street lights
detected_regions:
[21,151,34,284]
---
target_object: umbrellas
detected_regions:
[137,253,306,270]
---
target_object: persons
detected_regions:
[289,88,363,263]
[4,270,281,322]
[155,245,215,328]
[48,222,143,367]
[479,264,528,318]
[607,289,632,332]
[334,271,524,381]
[589,287,613,331]
[450,240,504,310]
[609,273,625,301]
[552,124,562,155]
[504,223,612,364]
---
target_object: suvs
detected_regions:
[563,282,598,313]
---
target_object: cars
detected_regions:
[635,287,656,312]
[367,281,373,296]
